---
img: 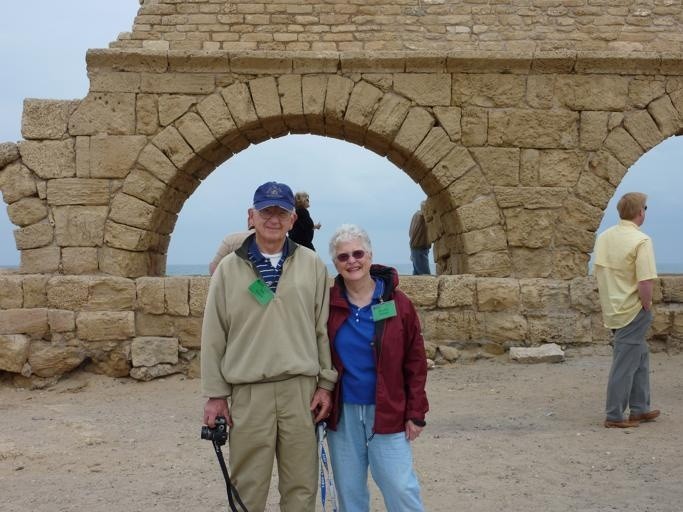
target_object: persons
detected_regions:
[407,198,431,276]
[321,223,430,512]
[591,191,661,429]
[287,191,322,252]
[207,212,256,278]
[195,178,341,511]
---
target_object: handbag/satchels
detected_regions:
[411,418,426,427]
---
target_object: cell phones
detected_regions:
[201,415,229,446]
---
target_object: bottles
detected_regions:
[253,181,294,213]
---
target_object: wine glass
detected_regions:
[326,412,330,418]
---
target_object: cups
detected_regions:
[337,250,364,261]
[254,208,290,221]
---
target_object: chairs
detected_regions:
[605,419,640,428]
[629,410,660,421]
